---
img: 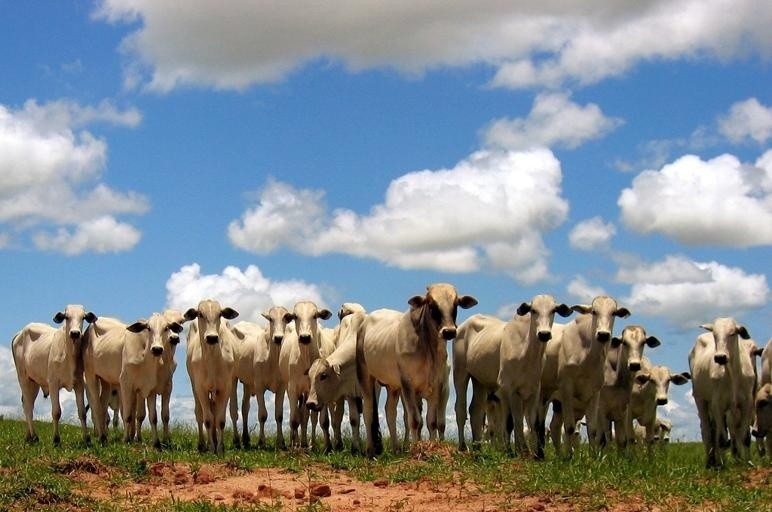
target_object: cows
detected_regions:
[230,306,294,449]
[279,283,688,462]
[681,317,772,470]
[12,304,183,450]
[183,300,239,458]
